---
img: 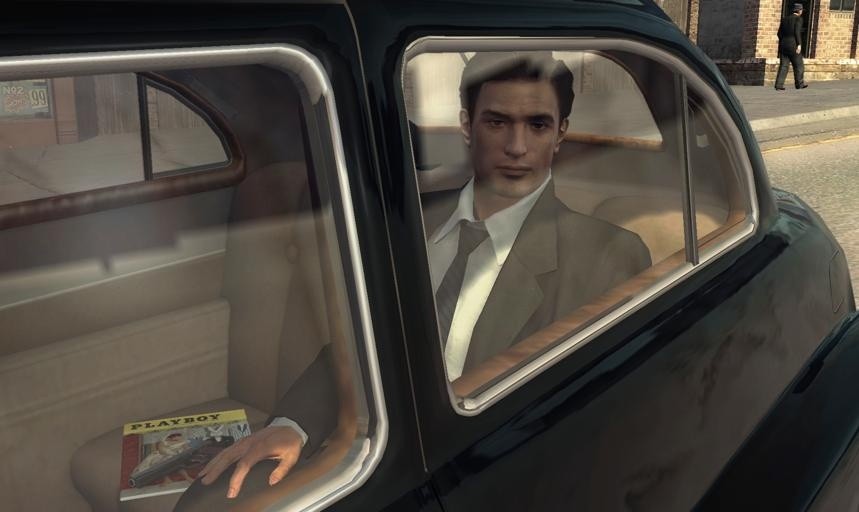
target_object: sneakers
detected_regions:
[794,83,809,89]
[774,86,786,91]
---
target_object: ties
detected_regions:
[435,216,493,361]
